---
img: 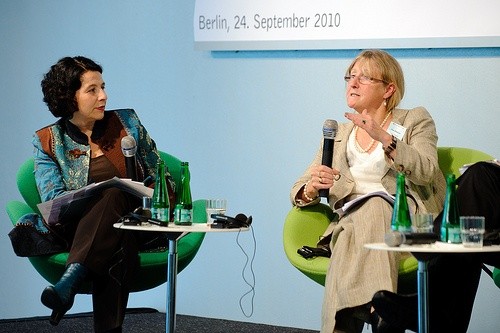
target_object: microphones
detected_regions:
[319,119,338,197]
[120,136,136,181]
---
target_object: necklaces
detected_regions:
[353,112,391,153]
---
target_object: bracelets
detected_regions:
[304,181,319,201]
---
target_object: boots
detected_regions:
[41,263,84,327]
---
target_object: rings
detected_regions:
[362,120,365,124]
[318,177,322,184]
[318,171,321,178]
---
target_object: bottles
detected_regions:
[440,174,463,244]
[150,160,171,227]
[391,172,414,244]
[173,161,194,225]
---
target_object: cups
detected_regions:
[414,213,435,234]
[205,199,227,228]
[459,216,485,247]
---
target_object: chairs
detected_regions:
[283,147,493,296]
[6,149,209,333]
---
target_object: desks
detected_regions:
[364,241,500,333]
[113,220,251,333]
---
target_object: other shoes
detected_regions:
[369,290,419,333]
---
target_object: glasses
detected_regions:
[344,73,389,85]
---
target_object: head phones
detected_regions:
[211,213,252,226]
[123,211,169,227]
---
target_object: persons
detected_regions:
[372,160,500,333]
[33,56,176,333]
[290,50,451,333]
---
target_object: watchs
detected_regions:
[382,135,397,159]
